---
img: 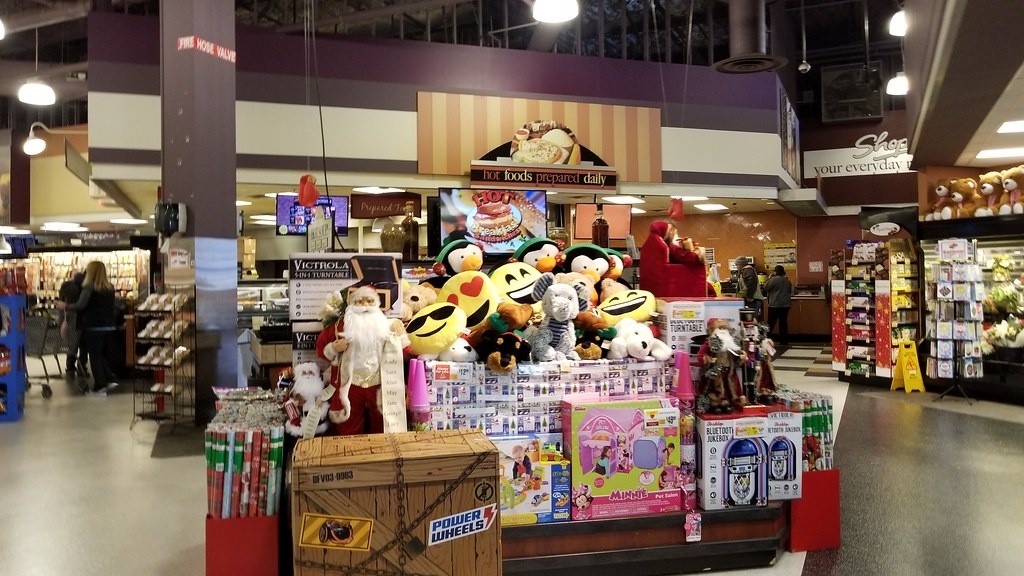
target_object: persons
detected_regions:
[280,360,333,438]
[314,284,414,435]
[761,265,793,344]
[511,445,534,480]
[676,468,681,475]
[593,447,613,479]
[659,471,667,489]
[52,260,124,397]
[699,318,750,408]
[736,308,779,405]
[734,255,766,334]
[662,443,674,468]
[650,221,703,266]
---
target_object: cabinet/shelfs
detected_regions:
[916,213,1024,410]
[129,282,196,436]
[0,294,25,422]
[837,221,924,390]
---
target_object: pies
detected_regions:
[519,138,562,164]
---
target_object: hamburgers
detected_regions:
[478,203,514,229]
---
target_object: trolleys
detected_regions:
[25,303,91,398]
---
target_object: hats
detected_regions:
[649,221,671,240]
[735,256,748,265]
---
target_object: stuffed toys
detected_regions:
[392,229,673,375]
[919,164,1024,221]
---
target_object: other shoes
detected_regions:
[107,382,119,392]
[66,369,75,378]
[85,388,107,397]
[77,369,90,377]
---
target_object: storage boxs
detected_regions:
[206,511,280,576]
[237,235,258,281]
[292,429,501,575]
[655,296,746,398]
[250,331,293,365]
[787,470,841,553]
[486,391,802,525]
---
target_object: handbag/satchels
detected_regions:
[60,320,69,338]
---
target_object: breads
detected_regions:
[139,293,190,366]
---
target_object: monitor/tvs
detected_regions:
[276,192,350,237]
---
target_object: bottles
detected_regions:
[401,201,419,262]
[592,211,609,248]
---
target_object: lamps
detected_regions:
[17,27,56,106]
[532,0,578,23]
[888,10,907,37]
[886,72,909,95]
[23,122,88,156]
